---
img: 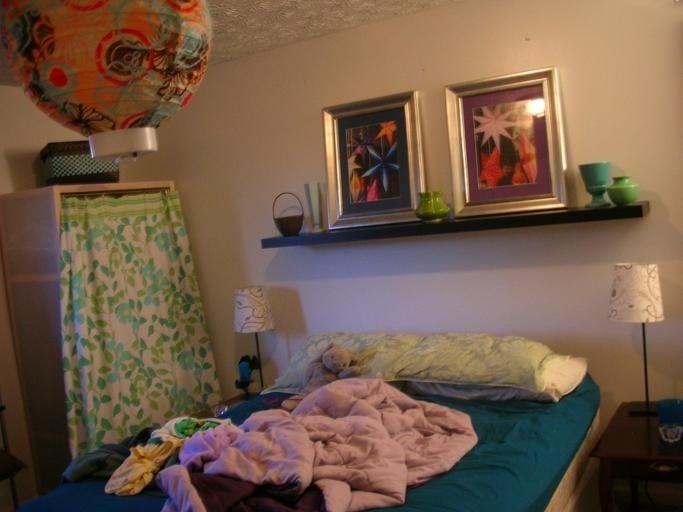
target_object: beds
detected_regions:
[32,369,606,508]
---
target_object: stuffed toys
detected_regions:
[281,342,361,411]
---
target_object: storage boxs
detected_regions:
[33,144,124,184]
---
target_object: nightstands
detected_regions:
[591,402,683,511]
[203,393,263,418]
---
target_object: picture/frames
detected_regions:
[320,90,426,230]
[444,65,568,218]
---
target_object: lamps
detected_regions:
[233,286,275,391]
[607,261,664,415]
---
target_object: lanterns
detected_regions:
[3,0,213,163]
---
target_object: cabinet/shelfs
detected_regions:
[1,182,177,493]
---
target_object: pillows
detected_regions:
[413,357,590,405]
[270,334,568,392]
